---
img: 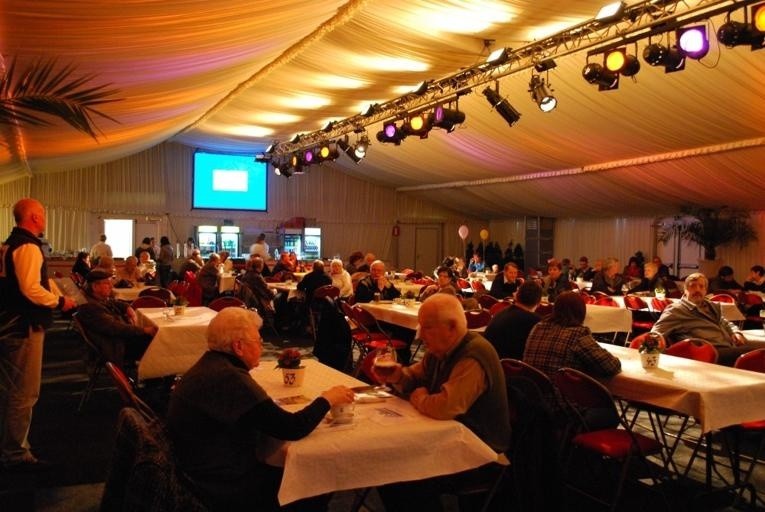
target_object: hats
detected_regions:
[87,270,113,283]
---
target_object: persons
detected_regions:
[94,255,115,273]
[172,250,205,281]
[365,253,377,268]
[183,237,198,259]
[328,259,354,298]
[219,251,230,264]
[354,259,401,303]
[490,262,521,300]
[61,270,158,375]
[350,251,369,273]
[134,237,156,266]
[419,252,499,301]
[249,254,274,275]
[649,273,746,366]
[136,252,156,275]
[198,253,224,299]
[68,251,93,296]
[559,259,572,281]
[526,263,543,290]
[36,233,52,262]
[583,261,602,281]
[742,266,765,293]
[623,257,641,277]
[574,256,591,280]
[710,266,743,292]
[270,250,299,278]
[523,292,621,444]
[239,255,277,319]
[0,199,77,461]
[547,260,579,303]
[371,293,512,512]
[113,256,139,284]
[484,281,543,359]
[166,306,355,511]
[156,236,174,265]
[296,255,333,304]
[589,259,622,295]
[149,237,161,261]
[250,233,271,276]
[89,235,112,264]
[650,256,670,287]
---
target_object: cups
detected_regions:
[374,293,380,305]
[330,402,355,423]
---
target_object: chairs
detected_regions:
[106,361,154,420]
[741,294,765,330]
[552,367,664,511]
[121,407,196,512]
[339,298,387,365]
[625,332,666,458]
[624,294,654,346]
[717,348,765,480]
[76,313,144,413]
[138,287,176,304]
[353,306,407,365]
[667,336,715,477]
[457,277,470,289]
[301,286,339,340]
[501,358,568,505]
[581,288,621,343]
[448,385,534,512]
[710,294,734,303]
[471,281,485,291]
[131,296,167,309]
[208,296,246,312]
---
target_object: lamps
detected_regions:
[642,42,686,73]
[717,20,765,53]
[605,47,639,77]
[582,62,620,91]
[355,136,370,159]
[360,104,375,117]
[480,86,522,127]
[403,114,429,139]
[412,81,428,95]
[677,28,710,60]
[527,76,557,114]
[433,105,465,125]
[334,138,363,165]
[594,0,626,24]
[323,120,333,131]
[290,134,301,143]
[265,142,333,177]
[486,46,508,64]
[377,121,401,147]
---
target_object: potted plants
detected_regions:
[653,204,759,280]
[172,296,189,315]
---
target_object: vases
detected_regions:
[640,349,658,369]
[282,365,305,388]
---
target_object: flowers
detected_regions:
[639,333,657,353]
[274,349,301,368]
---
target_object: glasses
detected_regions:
[239,336,264,345]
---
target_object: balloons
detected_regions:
[458,225,469,241]
[480,229,489,241]
